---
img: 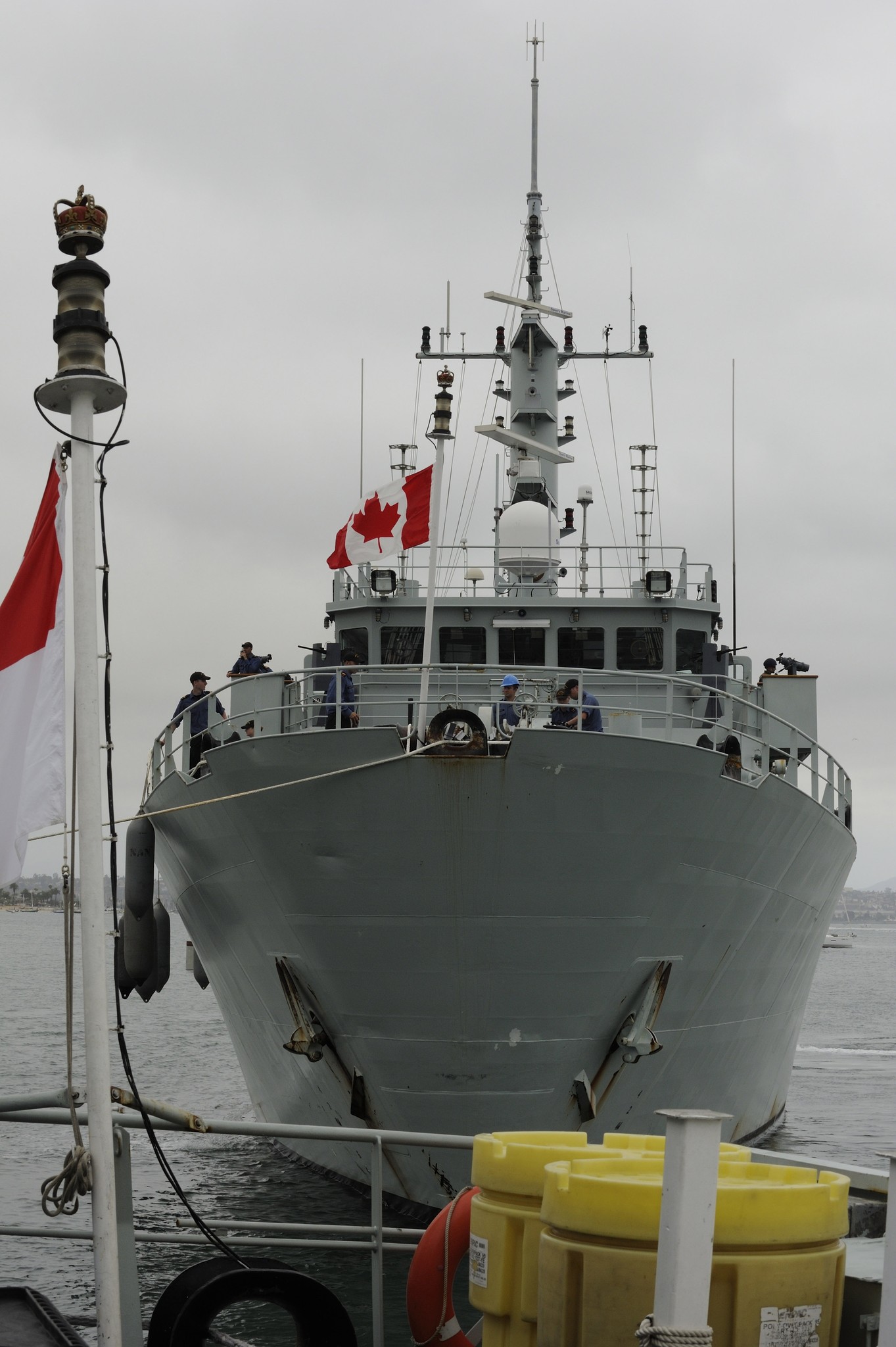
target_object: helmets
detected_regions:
[500,675,520,687]
[763,658,777,666]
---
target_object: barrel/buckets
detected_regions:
[468,1128,753,1346]
[533,1155,850,1346]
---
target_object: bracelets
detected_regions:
[566,722,570,727]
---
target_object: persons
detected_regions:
[759,658,777,682]
[492,675,531,755]
[241,720,254,737]
[550,689,579,726]
[562,679,602,733]
[226,642,260,677]
[161,672,227,779]
[325,652,365,730]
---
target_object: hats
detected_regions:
[343,652,365,664]
[241,720,254,729]
[556,688,569,700]
[242,642,253,648]
[190,672,211,683]
[562,679,579,695]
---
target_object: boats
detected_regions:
[105,906,125,914]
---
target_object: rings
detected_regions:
[243,654,243,655]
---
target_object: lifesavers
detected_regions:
[406,1185,482,1347]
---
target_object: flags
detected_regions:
[0,444,67,893]
[327,462,433,570]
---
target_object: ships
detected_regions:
[105,35,863,1205]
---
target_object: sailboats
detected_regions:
[52,900,65,913]
[822,893,857,948]
[74,896,81,913]
[5,890,40,912]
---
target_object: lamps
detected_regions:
[712,629,719,642]
[646,570,671,594]
[464,608,472,621]
[375,608,382,622]
[371,568,396,597]
[323,615,333,629]
[572,608,579,622]
[716,616,723,629]
[661,608,668,623]
